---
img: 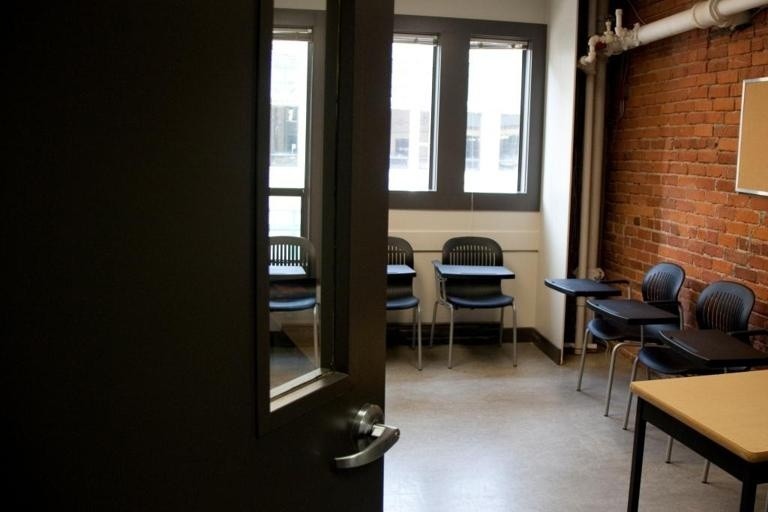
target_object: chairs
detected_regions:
[384,232,519,371]
[268,235,322,378]
[585,280,756,429]
[656,326,767,483]
[544,260,686,417]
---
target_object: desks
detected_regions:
[625,366,767,512]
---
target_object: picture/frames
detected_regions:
[735,76,768,200]
